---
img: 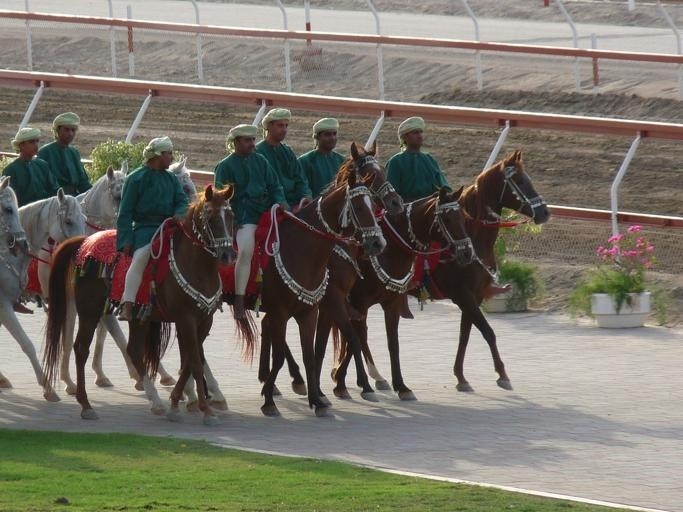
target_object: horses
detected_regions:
[0,138,476,426]
[329,148,551,393]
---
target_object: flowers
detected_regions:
[572,222,668,327]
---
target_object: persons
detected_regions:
[383,116,512,320]
[296,117,346,199]
[38,112,93,196]
[13,302,33,313]
[216,124,292,320]
[255,108,366,322]
[2,128,59,208]
[116,136,189,321]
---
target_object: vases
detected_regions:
[590,292,650,327]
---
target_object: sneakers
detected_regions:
[234,296,246,320]
[118,303,134,321]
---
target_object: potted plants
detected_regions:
[480,206,542,312]
